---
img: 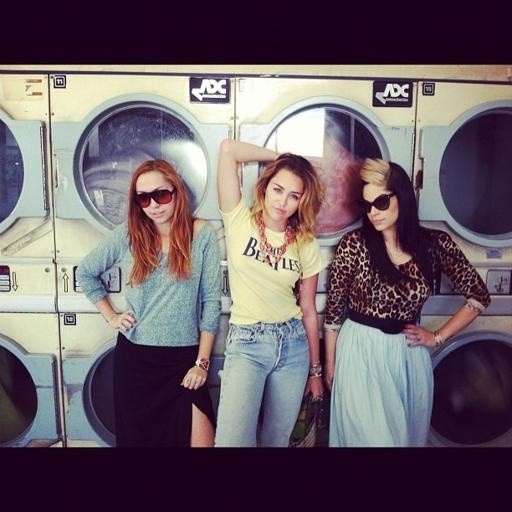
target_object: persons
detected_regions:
[213,137,327,448]
[324,156,491,446]
[74,158,217,449]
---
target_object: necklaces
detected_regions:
[254,211,296,264]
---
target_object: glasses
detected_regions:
[358,192,396,213]
[133,187,176,208]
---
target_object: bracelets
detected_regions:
[434,330,443,347]
[309,360,323,379]
[195,357,210,371]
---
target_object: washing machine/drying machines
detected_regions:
[1,63,512,447]
